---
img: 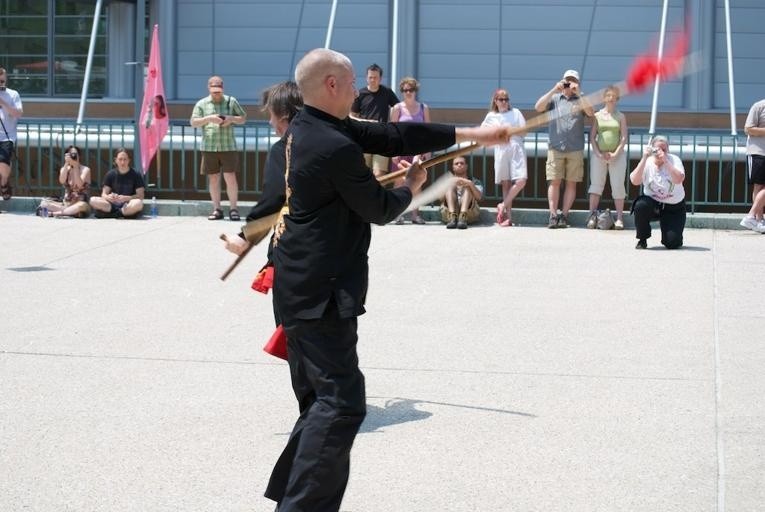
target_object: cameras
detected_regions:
[564,83,570,88]
[70,153,77,160]
[0,86,6,90]
[651,148,660,154]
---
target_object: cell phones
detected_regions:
[219,116,225,120]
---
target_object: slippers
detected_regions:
[412,214,425,225]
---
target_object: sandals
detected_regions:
[208,209,223,219]
[496,203,512,226]
[229,209,240,221]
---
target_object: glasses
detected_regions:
[498,98,508,102]
[403,88,415,92]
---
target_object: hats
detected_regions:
[563,70,579,81]
[208,76,223,93]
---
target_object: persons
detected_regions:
[739,99,765,233]
[225,81,304,361]
[440,156,485,229]
[534,70,594,228]
[390,77,432,225]
[265,47,510,512]
[0,67,23,201]
[89,147,145,219]
[36,146,92,218]
[190,76,246,221]
[629,135,686,248]
[586,85,629,230]
[349,64,401,189]
[480,88,528,226]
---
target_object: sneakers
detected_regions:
[447,212,467,228]
[615,218,624,230]
[586,211,597,229]
[740,214,765,233]
[548,212,567,229]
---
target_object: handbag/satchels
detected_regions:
[597,209,614,230]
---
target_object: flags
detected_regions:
[138,25,169,175]
